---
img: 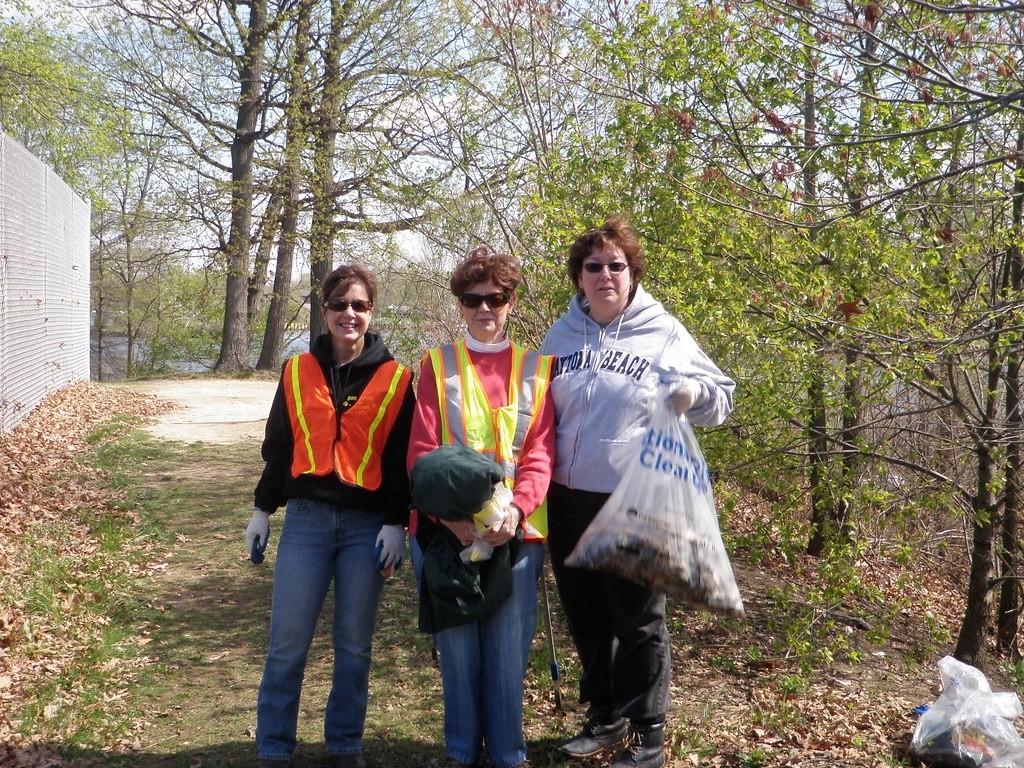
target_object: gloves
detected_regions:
[245,508,270,564]
[374,524,407,574]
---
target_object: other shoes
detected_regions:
[607,721,665,768]
[443,757,479,768]
[332,754,366,768]
[513,759,533,768]
[260,759,291,768]
[558,708,627,757]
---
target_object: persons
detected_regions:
[536,215,737,768]
[406,249,556,768]
[244,263,416,768]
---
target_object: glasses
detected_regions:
[323,299,372,313]
[583,263,629,272]
[460,292,510,308]
